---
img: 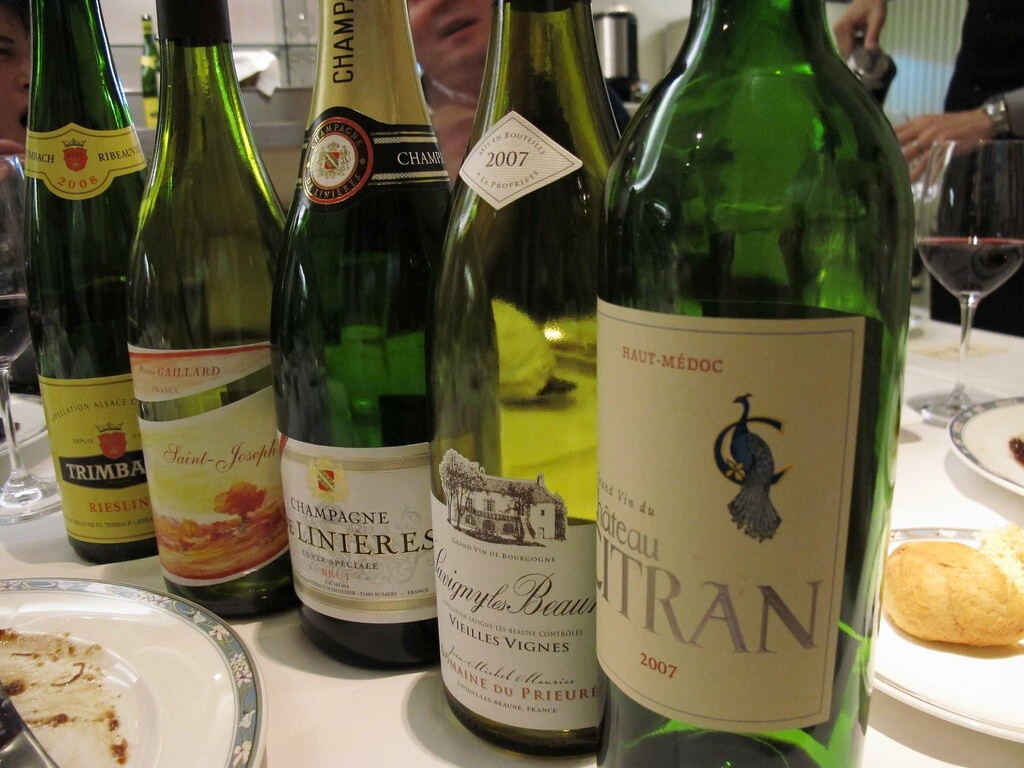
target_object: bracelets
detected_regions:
[983,95,1009,133]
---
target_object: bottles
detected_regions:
[139,14,162,130]
[24,0,150,565]
[122,0,292,626]
[272,0,502,672]
[592,10,639,97]
[420,0,624,758]
[591,0,917,768]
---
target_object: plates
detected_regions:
[0,576,269,768]
[871,524,1024,746]
[946,395,1024,497]
[0,392,48,457]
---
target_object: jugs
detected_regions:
[842,41,898,103]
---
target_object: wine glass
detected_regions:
[0,155,63,523]
[905,138,1024,427]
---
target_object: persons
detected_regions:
[336,0,674,191]
[0,0,45,393]
[833,1,1024,335]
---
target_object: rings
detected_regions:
[913,141,923,155]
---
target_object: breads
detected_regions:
[886,521,1024,647]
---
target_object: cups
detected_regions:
[887,112,938,203]
